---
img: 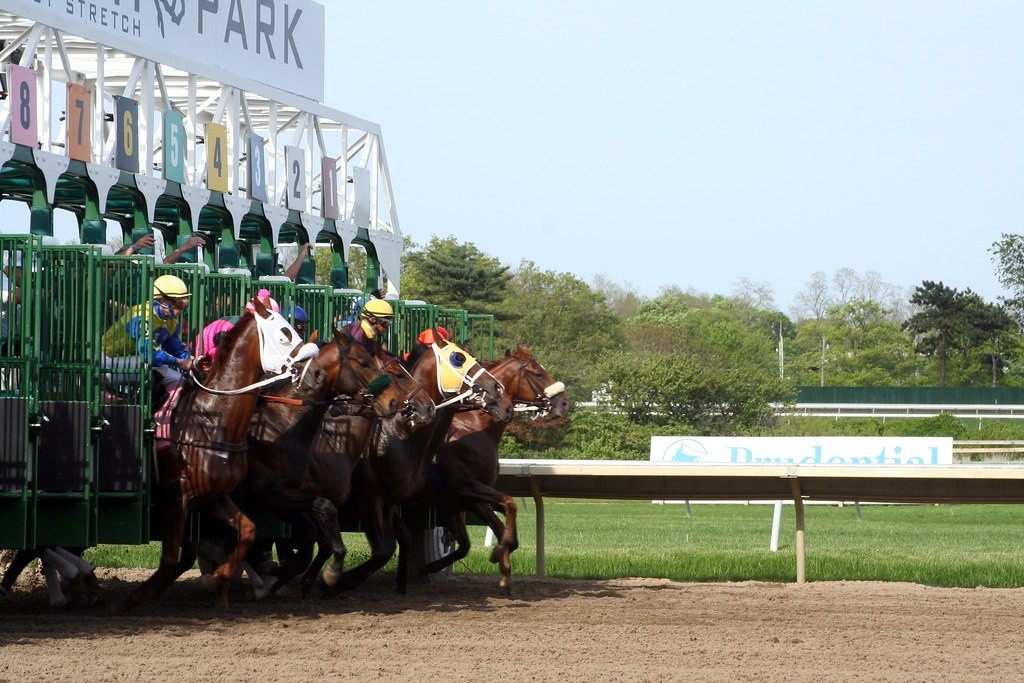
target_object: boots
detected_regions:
[124,371,163,409]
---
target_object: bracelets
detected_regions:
[130,246,138,252]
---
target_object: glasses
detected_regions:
[378,319,392,328]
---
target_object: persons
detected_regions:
[0,232,389,402]
[101,274,196,446]
[403,328,443,363]
[183,287,279,363]
[307,297,394,358]
[280,306,308,338]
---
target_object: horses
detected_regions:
[0,292,571,619]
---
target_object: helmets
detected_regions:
[153,274,193,299]
[418,329,444,347]
[281,305,309,323]
[361,299,395,317]
